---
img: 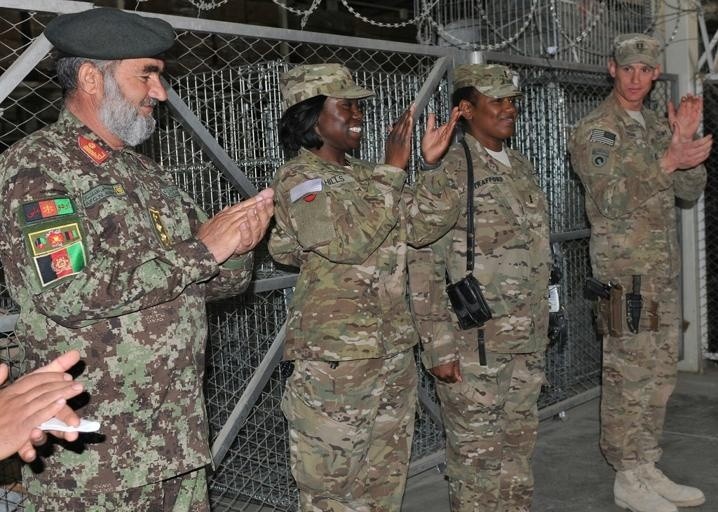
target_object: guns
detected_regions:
[549,309,566,353]
[584,277,623,337]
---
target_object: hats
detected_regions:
[44,8,175,60]
[614,32,661,69]
[280,64,376,113]
[453,64,524,100]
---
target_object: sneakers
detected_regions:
[640,462,705,507]
[614,468,678,512]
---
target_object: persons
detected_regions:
[0,7,275,512]
[566,33,714,512]
[0,348,88,463]
[405,63,553,512]
[266,62,463,511]
